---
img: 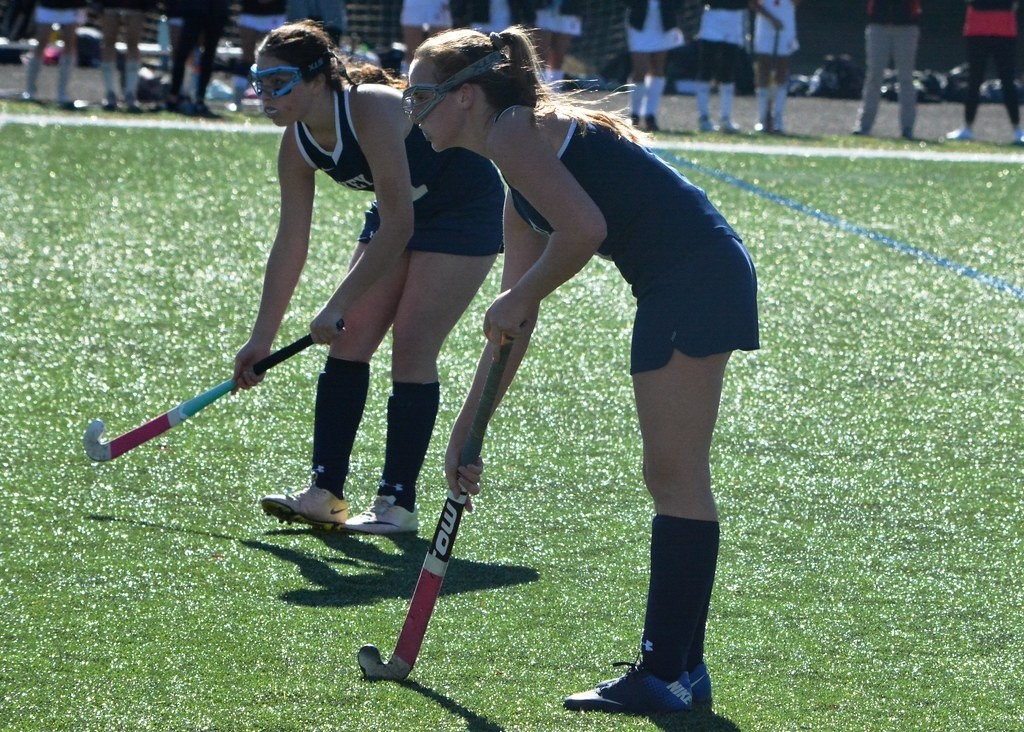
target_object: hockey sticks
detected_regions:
[358,326,517,681]
[762,24,779,133]
[83,318,353,461]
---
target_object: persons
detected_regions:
[751,0,800,133]
[399,0,587,92]
[947,0,1024,143]
[852,0,921,141]
[95,0,289,116]
[231,20,505,536]
[624,0,684,130]
[695,0,756,132]
[407,26,760,714]
[25,0,89,109]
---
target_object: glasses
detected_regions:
[401,83,446,124]
[249,64,302,98]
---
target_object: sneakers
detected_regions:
[342,495,421,535]
[689,661,712,704]
[563,653,692,715]
[262,484,349,531]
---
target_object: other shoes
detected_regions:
[718,119,740,132]
[770,120,783,133]
[22,86,37,100]
[754,116,769,132]
[630,114,641,129]
[58,97,76,110]
[646,114,659,132]
[103,93,116,111]
[126,100,141,113]
[194,101,222,118]
[164,95,199,116]
[1013,128,1024,145]
[852,123,870,136]
[902,127,914,140]
[946,127,976,140]
[698,116,713,132]
